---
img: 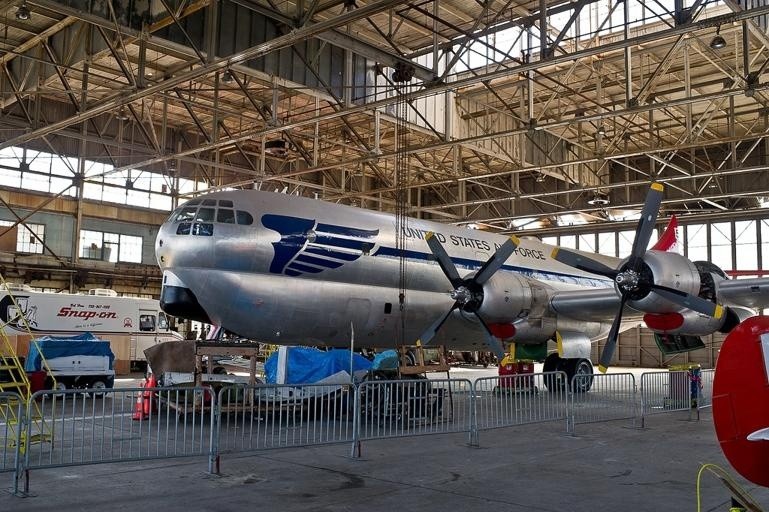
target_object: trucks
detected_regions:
[0,282,182,371]
[24,333,115,399]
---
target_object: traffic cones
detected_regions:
[132,378,154,420]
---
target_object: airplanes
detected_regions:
[155,184,769,394]
